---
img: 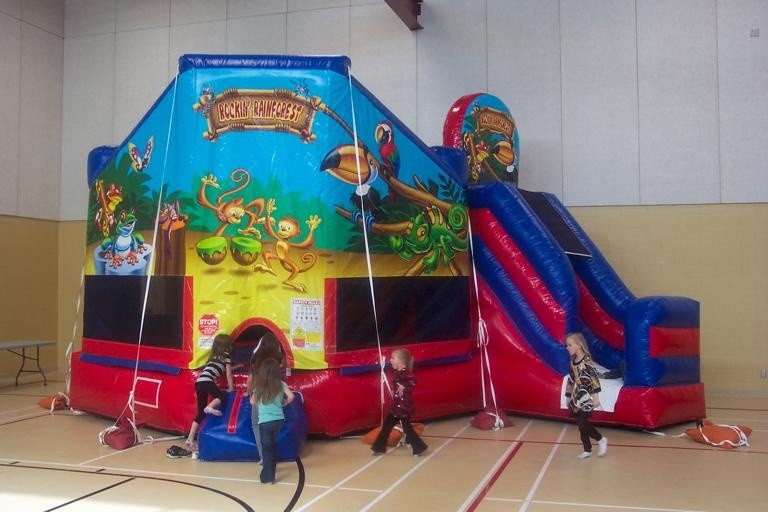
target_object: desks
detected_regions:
[0,340,57,386]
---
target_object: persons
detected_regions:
[368,348,429,456]
[253,327,283,354]
[563,330,609,457]
[184,332,234,453]
[244,333,287,466]
[250,356,296,485]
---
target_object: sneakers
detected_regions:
[413,447,430,457]
[598,437,608,456]
[166,445,199,459]
[368,450,386,455]
[575,451,592,459]
